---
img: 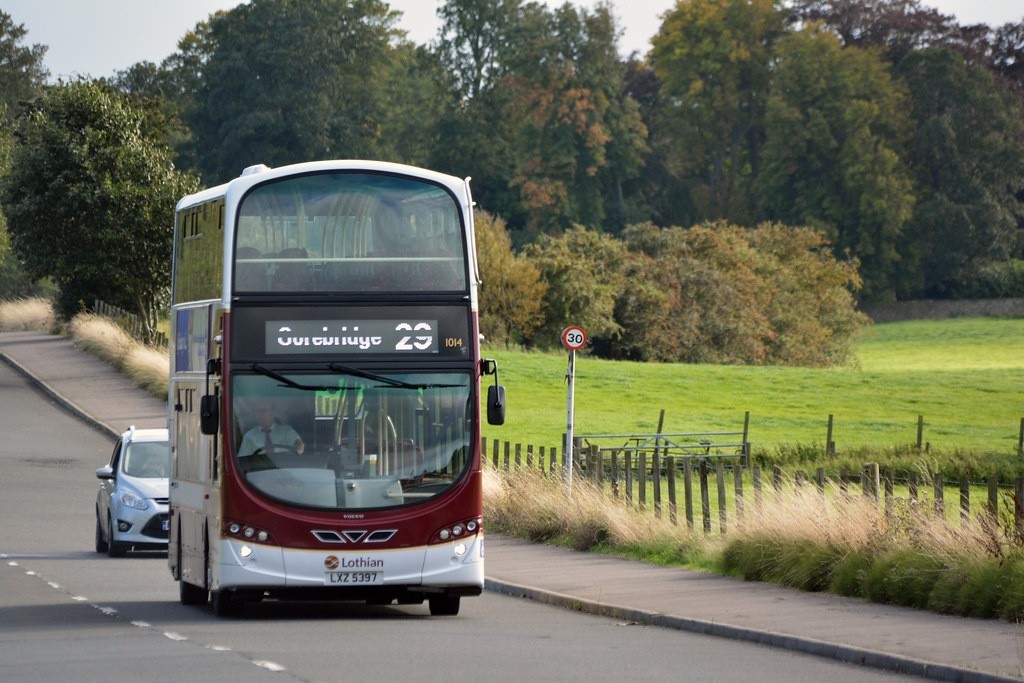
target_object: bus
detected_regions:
[163,156,507,617]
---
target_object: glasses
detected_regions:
[255,408,272,414]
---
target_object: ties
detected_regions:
[260,428,278,464]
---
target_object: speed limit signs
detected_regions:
[561,326,587,350]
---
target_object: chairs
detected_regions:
[369,251,415,294]
[316,255,367,292]
[274,248,317,294]
[417,249,461,293]
[234,247,268,291]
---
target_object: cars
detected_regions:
[93,425,171,558]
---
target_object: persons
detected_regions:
[235,401,306,457]
[143,453,163,476]
